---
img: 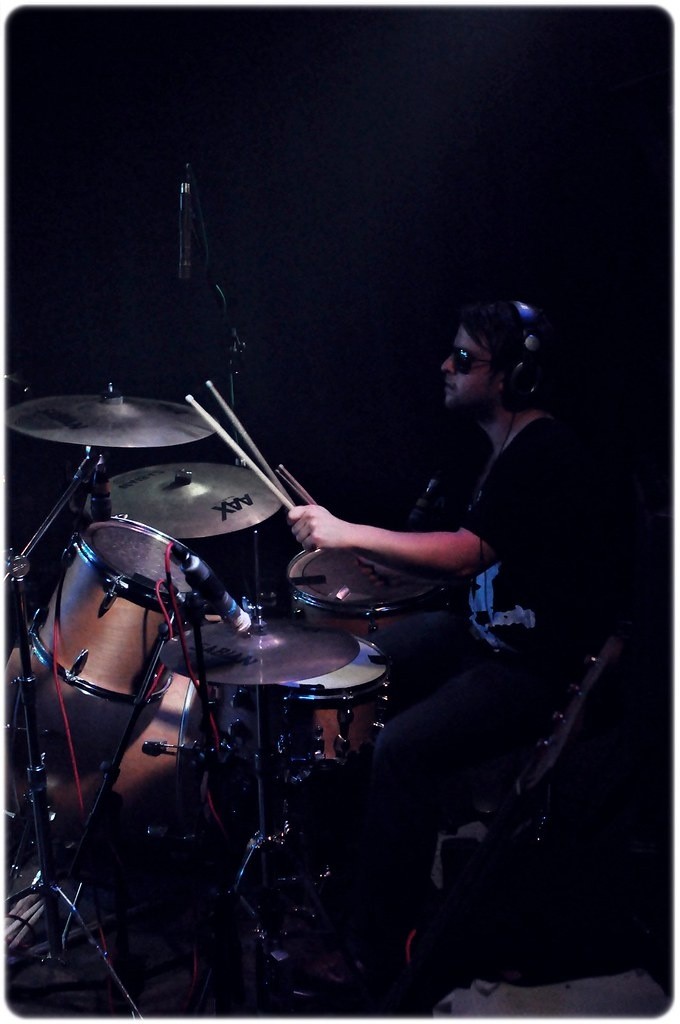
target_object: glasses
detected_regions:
[444,342,503,375]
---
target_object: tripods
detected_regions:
[8,445,380,1015]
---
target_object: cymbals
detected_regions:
[6,397,214,449]
[97,462,287,540]
[161,618,360,686]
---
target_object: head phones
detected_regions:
[506,300,544,401]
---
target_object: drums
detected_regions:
[287,542,460,677]
[8,627,217,835]
[279,637,392,762]
[27,518,206,702]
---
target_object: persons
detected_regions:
[288,298,586,986]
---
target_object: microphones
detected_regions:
[169,543,253,632]
[179,164,194,280]
[90,457,113,522]
[253,530,276,606]
[409,476,440,525]
